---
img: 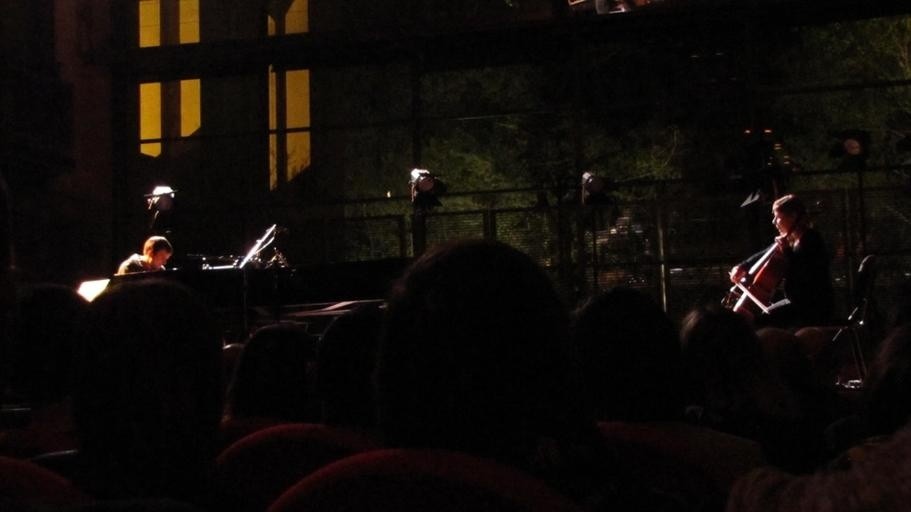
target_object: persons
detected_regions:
[729,194,843,323]
[3,240,909,510]
[117,234,178,275]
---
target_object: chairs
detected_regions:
[1,401,763,512]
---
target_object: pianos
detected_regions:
[76,252,241,305]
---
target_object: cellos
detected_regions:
[729,199,826,321]
